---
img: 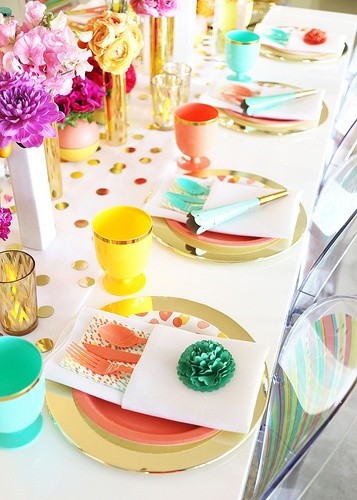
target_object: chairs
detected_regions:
[243,38,357,500]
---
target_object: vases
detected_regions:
[148,12,174,92]
[101,70,127,148]
[51,114,99,162]
[4,136,58,250]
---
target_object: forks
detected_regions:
[166,193,202,212]
[66,341,133,375]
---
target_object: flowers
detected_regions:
[177,339,238,394]
[0,207,11,242]
[0,0,178,157]
[303,28,326,45]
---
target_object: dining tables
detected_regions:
[0,0,357,499]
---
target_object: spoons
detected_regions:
[178,179,210,194]
[99,324,148,347]
[233,84,254,97]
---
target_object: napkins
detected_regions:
[199,181,301,243]
[120,323,268,434]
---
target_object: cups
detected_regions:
[163,62,192,105]
[0,250,39,337]
[151,73,180,131]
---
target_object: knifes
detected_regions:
[168,191,204,204]
[84,343,141,364]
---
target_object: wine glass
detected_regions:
[92,206,153,296]
[225,30,261,82]
[0,336,45,450]
[174,103,219,171]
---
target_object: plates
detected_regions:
[143,169,307,264]
[260,25,349,63]
[46,296,270,474]
[200,81,329,136]
